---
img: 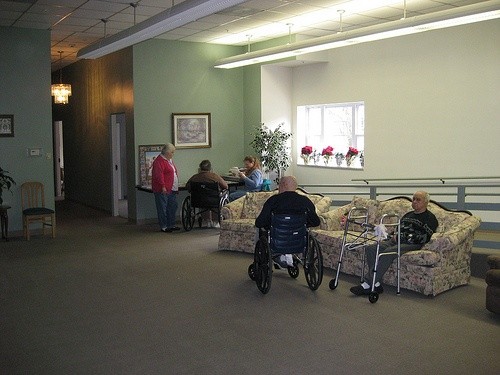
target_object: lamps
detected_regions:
[51,51,72,105]
[77,0,500,70]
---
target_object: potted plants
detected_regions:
[0,167,16,206]
[309,150,320,164]
[335,153,345,165]
[359,149,365,167]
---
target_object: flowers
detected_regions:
[300,146,314,160]
[321,145,335,157]
[345,147,359,159]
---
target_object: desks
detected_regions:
[0,205,11,242]
[136,181,243,194]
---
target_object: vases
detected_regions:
[303,155,309,165]
[323,155,331,165]
[345,155,356,167]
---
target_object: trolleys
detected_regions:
[330,207,400,304]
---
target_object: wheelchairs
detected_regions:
[181,188,229,231]
[247,222,323,294]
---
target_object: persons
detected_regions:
[186,160,228,228]
[255,176,321,270]
[350,191,439,295]
[152,143,181,232]
[229,156,263,202]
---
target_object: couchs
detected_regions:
[485,254,500,315]
[302,195,482,298]
[217,186,332,260]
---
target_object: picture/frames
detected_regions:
[0,114,14,137]
[171,112,212,150]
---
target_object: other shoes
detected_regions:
[160,226,172,232]
[350,283,384,296]
[170,225,180,231]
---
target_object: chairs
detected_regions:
[20,182,56,241]
